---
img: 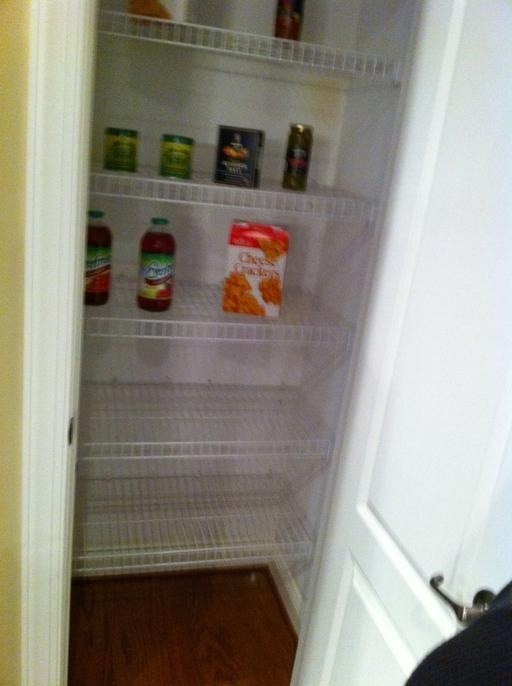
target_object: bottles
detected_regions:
[136,216,177,313]
[84,208,113,306]
[104,128,139,174]
[274,1,304,43]
[157,133,196,181]
[279,122,315,192]
